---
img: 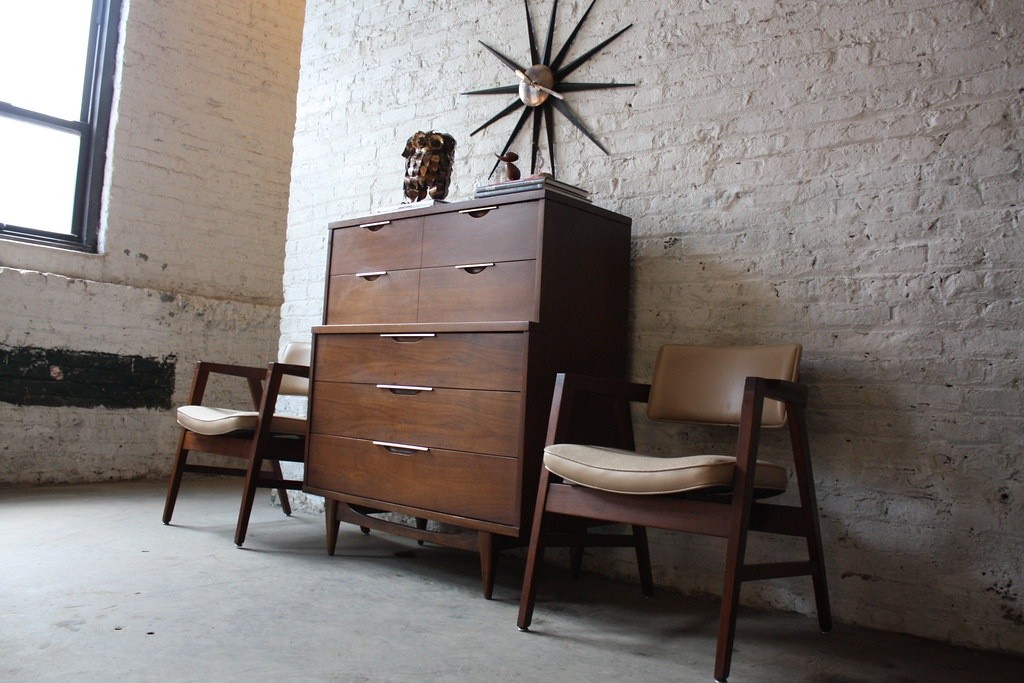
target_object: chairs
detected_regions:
[161,341,370,550]
[515,344,834,683]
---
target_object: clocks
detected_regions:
[459,0,635,181]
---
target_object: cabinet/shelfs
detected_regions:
[321,189,633,342]
[302,321,627,600]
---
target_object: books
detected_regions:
[474,177,593,205]
